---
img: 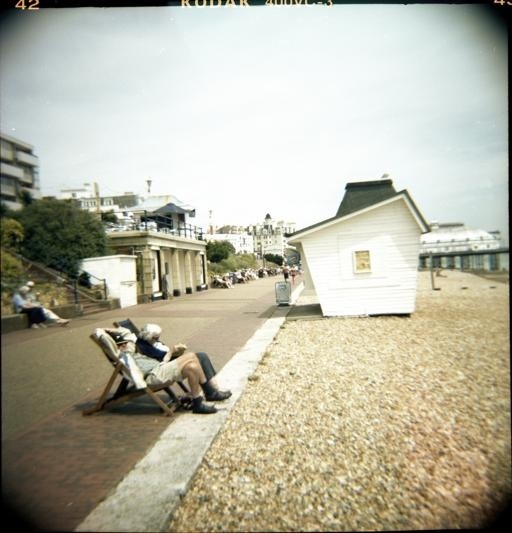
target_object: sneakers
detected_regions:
[31,319,70,328]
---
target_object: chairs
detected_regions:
[82,318,189,416]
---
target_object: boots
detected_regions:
[200,382,232,402]
[192,396,217,414]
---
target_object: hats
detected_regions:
[111,332,128,345]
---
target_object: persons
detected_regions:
[122,212,139,230]
[138,324,232,402]
[213,263,301,289]
[12,285,48,330]
[105,327,226,416]
[25,280,72,326]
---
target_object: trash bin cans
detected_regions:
[276,281,290,307]
